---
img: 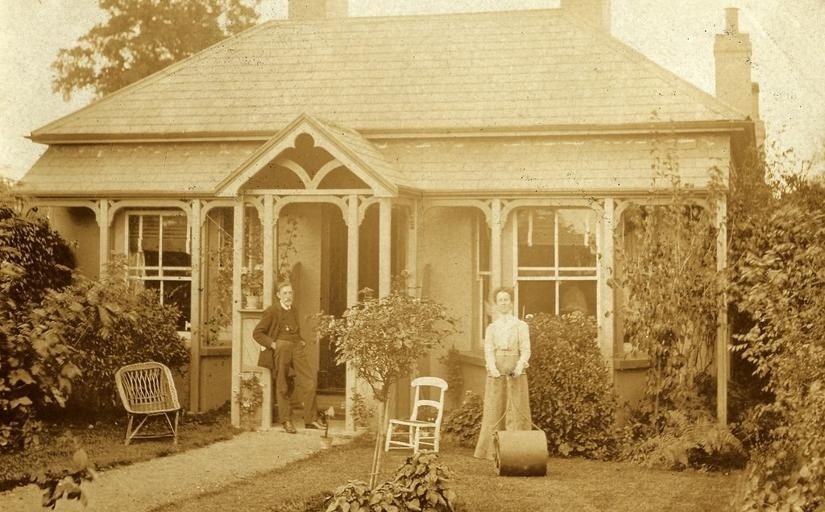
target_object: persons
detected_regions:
[473,285,533,461]
[252,281,329,435]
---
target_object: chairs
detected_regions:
[112,360,182,444]
[384,375,449,457]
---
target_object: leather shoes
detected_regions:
[283,420,297,433]
[304,420,327,430]
